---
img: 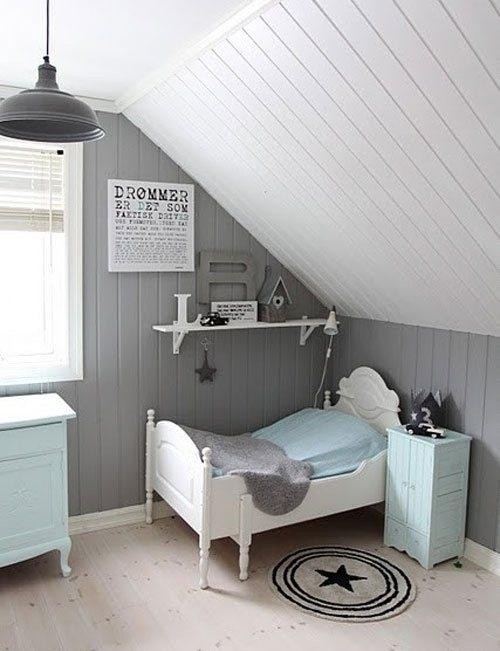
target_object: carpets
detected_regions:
[267,543,417,623]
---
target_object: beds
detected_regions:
[145,366,403,590]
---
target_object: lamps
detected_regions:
[323,305,339,335]
[0,0,107,143]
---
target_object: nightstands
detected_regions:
[383,423,473,570]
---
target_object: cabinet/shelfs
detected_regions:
[0,392,77,578]
